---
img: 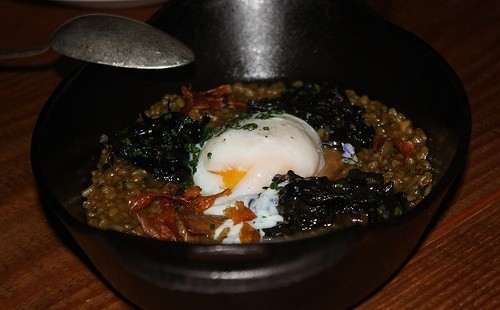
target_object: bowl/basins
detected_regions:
[29,1,473,309]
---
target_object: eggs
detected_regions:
[193,112,326,243]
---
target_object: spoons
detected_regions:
[0,14,195,69]
